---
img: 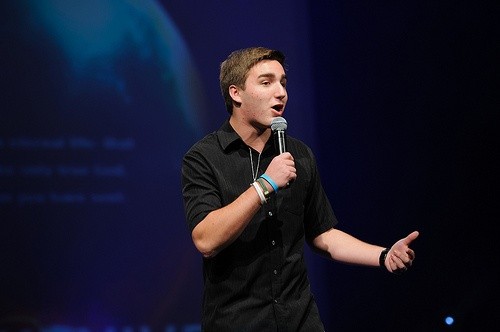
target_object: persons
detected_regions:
[177,44,421,332]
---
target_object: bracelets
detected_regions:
[256,178,271,200]
[378,248,390,267]
[252,181,268,205]
[259,173,278,192]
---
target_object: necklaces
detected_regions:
[247,147,263,183]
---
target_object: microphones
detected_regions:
[271,116,290,189]
